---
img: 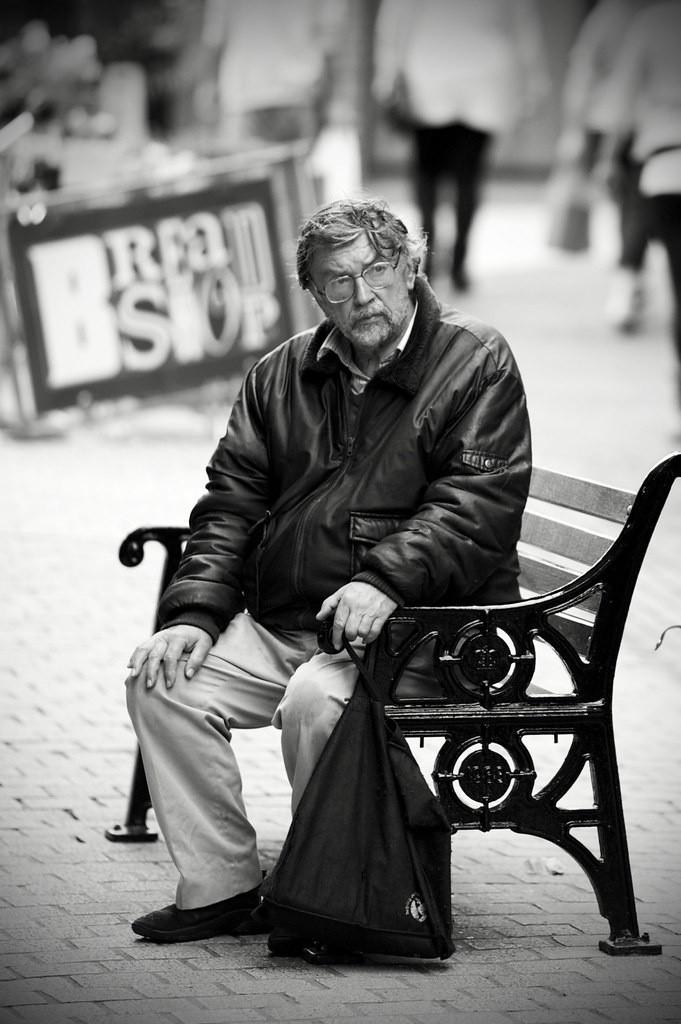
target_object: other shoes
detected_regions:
[133,869,267,942]
[267,909,328,950]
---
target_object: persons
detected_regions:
[178,0,681,409]
[122,199,539,962]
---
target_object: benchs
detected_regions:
[102,452,679,957]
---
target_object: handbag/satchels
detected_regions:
[262,636,458,960]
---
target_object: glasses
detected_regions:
[306,248,402,304]
